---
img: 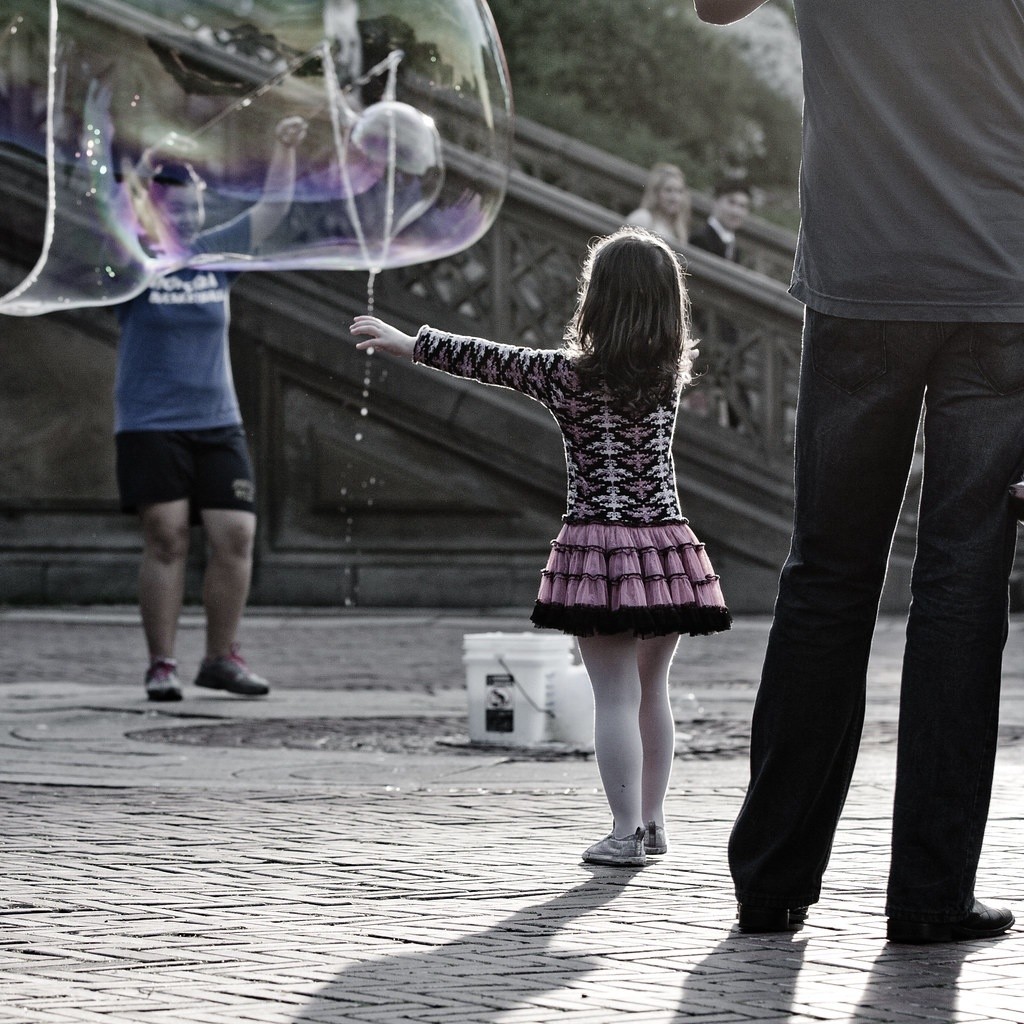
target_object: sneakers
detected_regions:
[145,657,182,701]
[195,642,270,696]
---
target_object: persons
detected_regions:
[101,118,309,700]
[627,163,691,248]
[690,0,1024,939]
[689,183,756,428]
[349,234,732,864]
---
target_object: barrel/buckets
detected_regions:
[462,631,571,745]
[553,664,595,747]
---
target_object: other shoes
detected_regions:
[887,900,1014,944]
[644,820,668,855]
[736,900,810,931]
[582,819,647,867]
